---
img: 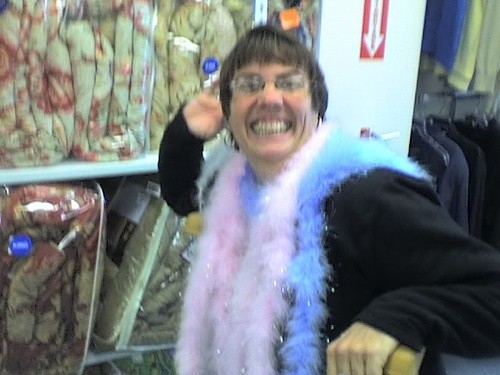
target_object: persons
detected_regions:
[158,25,500,375]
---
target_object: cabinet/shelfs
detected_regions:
[0,0,312,375]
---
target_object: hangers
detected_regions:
[412,89,500,162]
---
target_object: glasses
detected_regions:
[231,73,306,91]
